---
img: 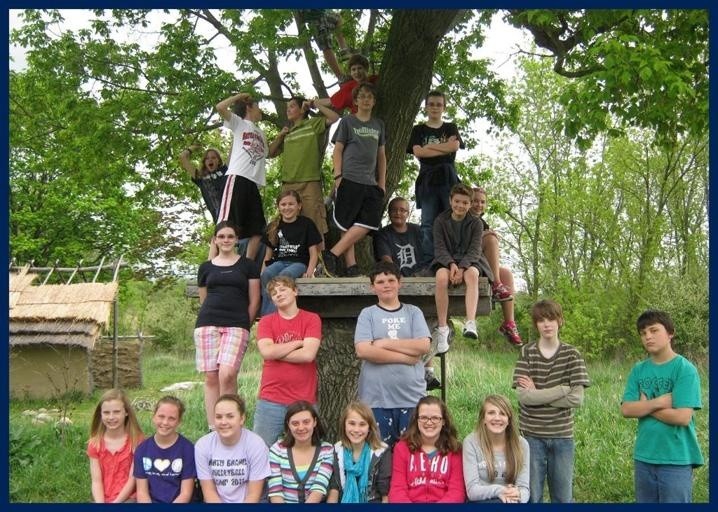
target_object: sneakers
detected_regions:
[424,370,440,391]
[462,318,478,340]
[491,282,513,302]
[498,320,524,349]
[434,323,450,354]
[317,248,338,279]
[344,265,366,279]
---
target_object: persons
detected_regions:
[327,403,391,503]
[254,276,322,447]
[193,394,270,503]
[86,388,144,503]
[620,310,705,503]
[461,394,531,504]
[352,260,432,504]
[190,221,261,432]
[133,395,197,504]
[512,299,590,503]
[387,396,465,504]
[267,399,335,503]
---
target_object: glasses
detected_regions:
[389,206,409,215]
[417,416,445,424]
[426,102,445,108]
